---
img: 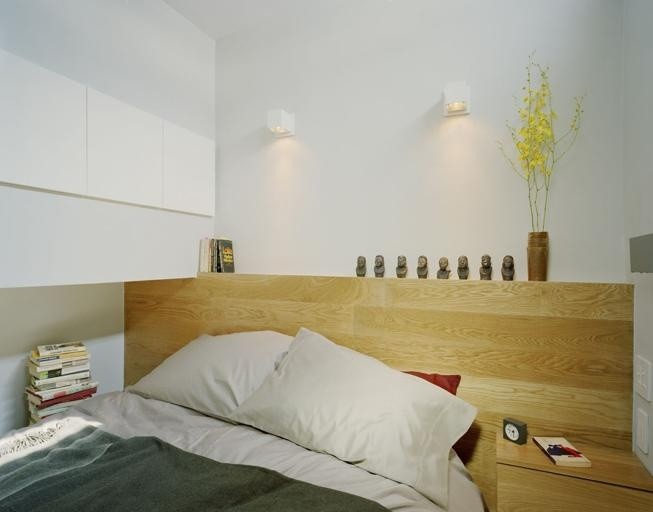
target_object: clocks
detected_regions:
[503,417,527,445]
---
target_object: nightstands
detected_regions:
[496,429,652,511]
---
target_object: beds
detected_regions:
[0,273,635,511]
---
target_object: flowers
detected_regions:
[497,51,585,231]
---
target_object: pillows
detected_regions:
[126,330,297,426]
[401,371,461,396]
[223,326,479,510]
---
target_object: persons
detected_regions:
[373,255,385,278]
[478,255,492,280]
[499,255,514,281]
[396,255,407,278]
[416,255,428,279]
[355,255,366,277]
[436,257,450,280]
[456,256,469,280]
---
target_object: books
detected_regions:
[22,339,98,428]
[531,435,591,468]
[197,236,234,273]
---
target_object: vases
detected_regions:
[527,231,549,281]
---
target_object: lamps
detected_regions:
[265,108,296,138]
[444,81,470,116]
